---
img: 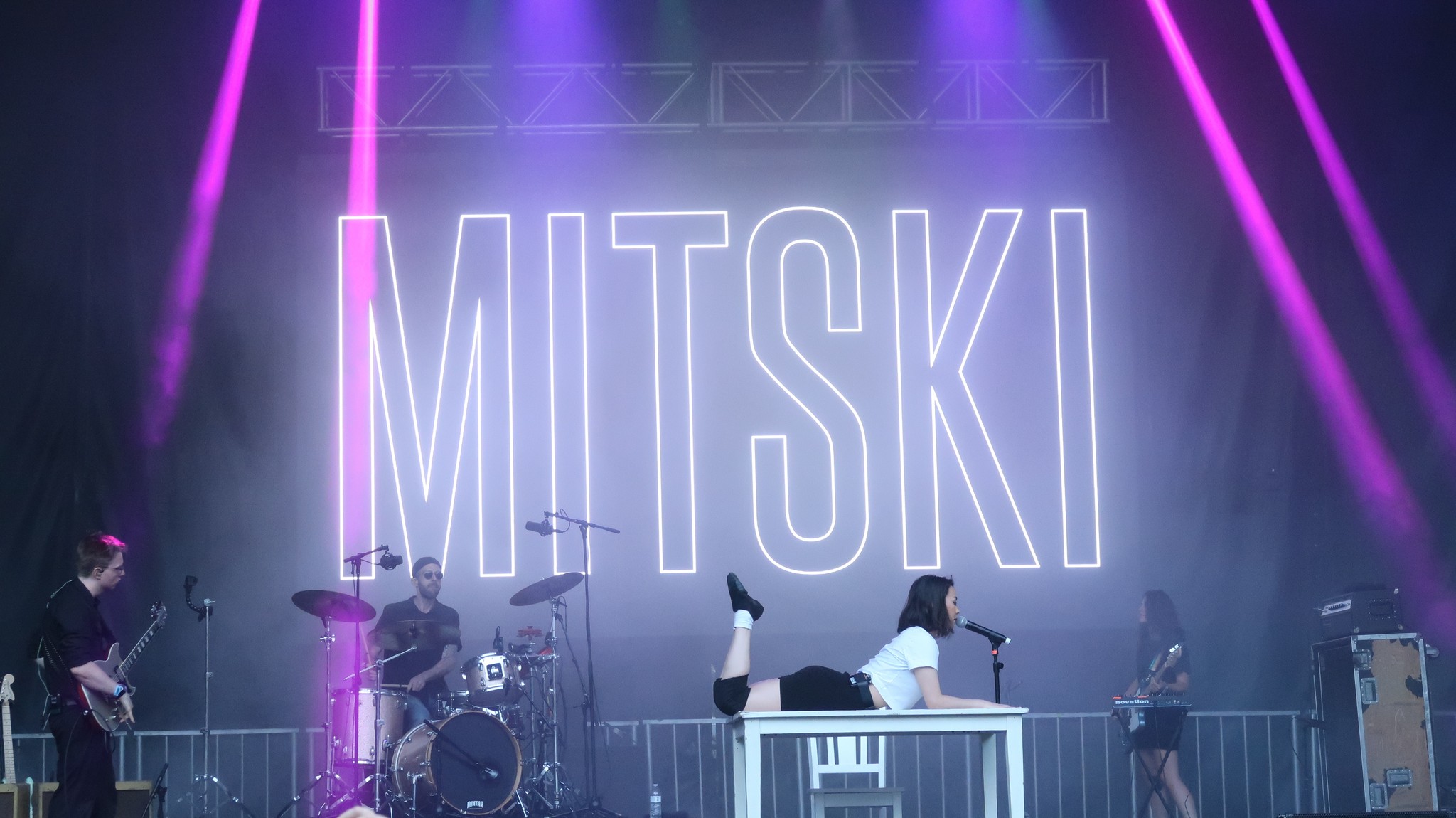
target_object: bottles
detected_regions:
[649,784,662,818]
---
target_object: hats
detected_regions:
[412,557,442,576]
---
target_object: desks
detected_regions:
[726,709,1027,818]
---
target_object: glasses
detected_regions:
[424,570,443,580]
[88,566,123,576]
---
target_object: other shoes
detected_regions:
[727,573,764,620]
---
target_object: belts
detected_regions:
[854,672,875,710]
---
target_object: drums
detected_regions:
[461,652,525,709]
[493,705,526,736]
[434,691,472,718]
[390,711,522,818]
[330,688,409,768]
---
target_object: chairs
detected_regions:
[808,734,905,818]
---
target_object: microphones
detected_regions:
[545,598,560,646]
[377,555,403,569]
[955,616,1010,646]
[524,521,559,536]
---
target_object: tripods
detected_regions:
[162,608,258,818]
[512,585,591,813]
[270,540,413,818]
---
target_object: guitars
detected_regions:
[0,674,34,818]
[77,600,168,733]
[1116,642,1185,755]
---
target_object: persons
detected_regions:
[361,557,463,731]
[713,572,1022,715]
[29,532,136,818]
[1111,589,1199,818]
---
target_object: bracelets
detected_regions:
[112,684,124,697]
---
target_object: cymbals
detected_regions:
[366,618,462,652]
[509,572,584,607]
[292,590,376,623]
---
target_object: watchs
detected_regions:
[117,685,128,698]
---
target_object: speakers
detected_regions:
[0,782,30,817]
[36,780,151,817]
[1318,589,1403,639]
